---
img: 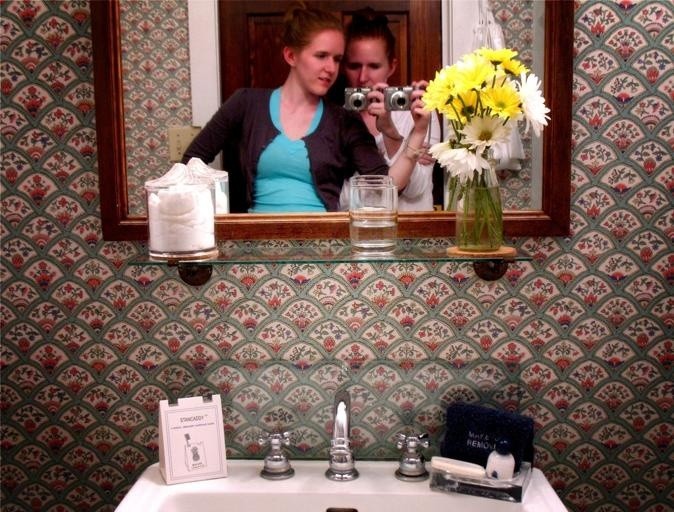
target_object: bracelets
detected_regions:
[406,144,419,154]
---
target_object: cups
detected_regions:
[349,175,393,213]
[144,177,220,262]
[166,171,231,215]
[350,185,399,254]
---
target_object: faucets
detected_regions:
[325,389,359,481]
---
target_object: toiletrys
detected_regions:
[484,437,516,479]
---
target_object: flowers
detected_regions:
[421,47,551,248]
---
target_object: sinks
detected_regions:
[115,458,569,512]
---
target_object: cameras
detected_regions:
[343,88,374,111]
[384,86,414,111]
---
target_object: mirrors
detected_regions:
[89,0,575,241]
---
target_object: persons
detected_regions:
[178,1,433,214]
[338,4,442,212]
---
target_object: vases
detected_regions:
[456,185,504,252]
